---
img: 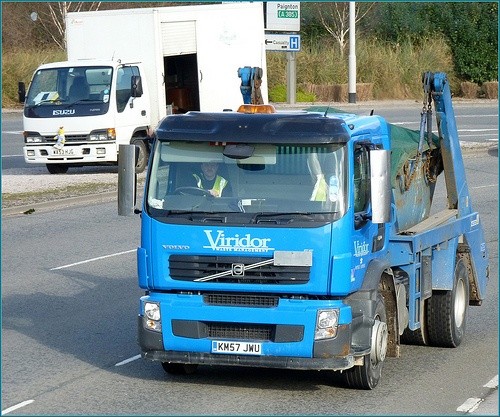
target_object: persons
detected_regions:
[190,162,232,197]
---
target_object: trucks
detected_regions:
[116,65,491,394]
[17,2,270,174]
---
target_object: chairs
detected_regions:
[68,76,90,100]
[217,169,234,198]
